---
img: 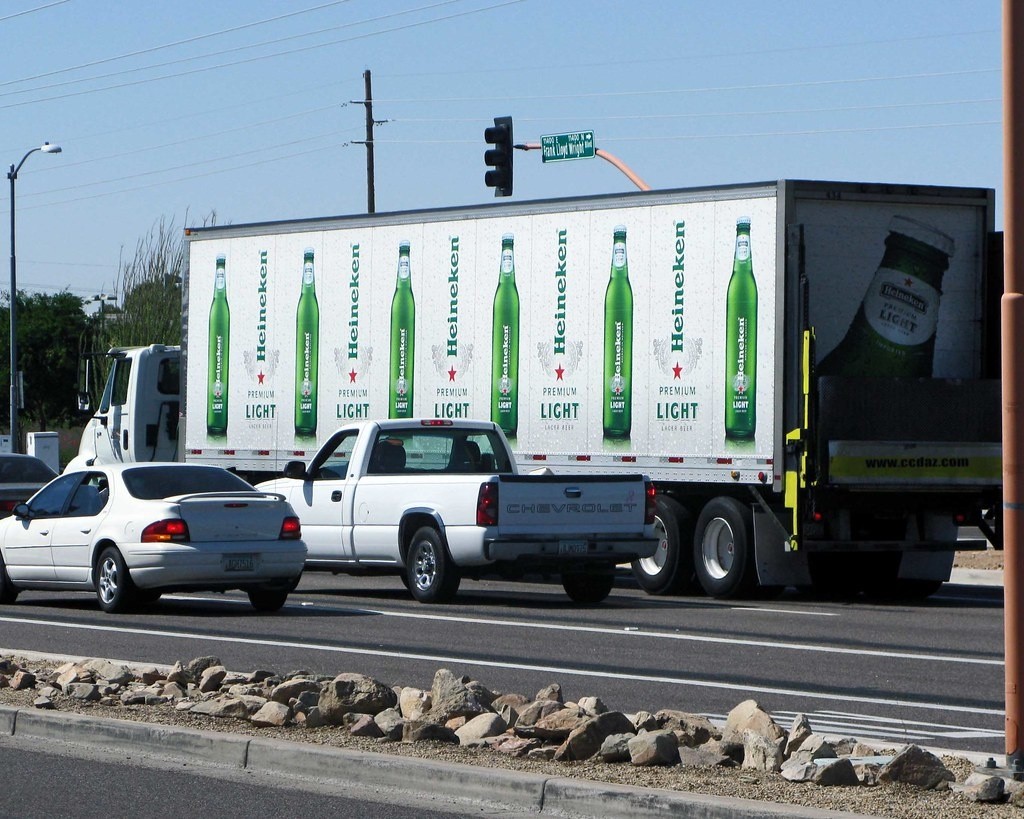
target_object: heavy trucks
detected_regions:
[63,176,1007,603]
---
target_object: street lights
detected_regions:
[7,142,64,454]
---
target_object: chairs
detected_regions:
[369,441,407,471]
[447,441,481,469]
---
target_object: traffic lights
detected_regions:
[485,114,513,196]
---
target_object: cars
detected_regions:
[0,462,309,616]
[0,452,58,519]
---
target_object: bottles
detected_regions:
[491,233,520,436]
[296,247,318,435]
[389,240,415,420]
[206,254,230,434]
[603,224,633,437]
[726,216,758,438]
[816,214,957,379]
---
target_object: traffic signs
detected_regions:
[541,130,596,163]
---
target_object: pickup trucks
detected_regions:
[254,416,661,608]
